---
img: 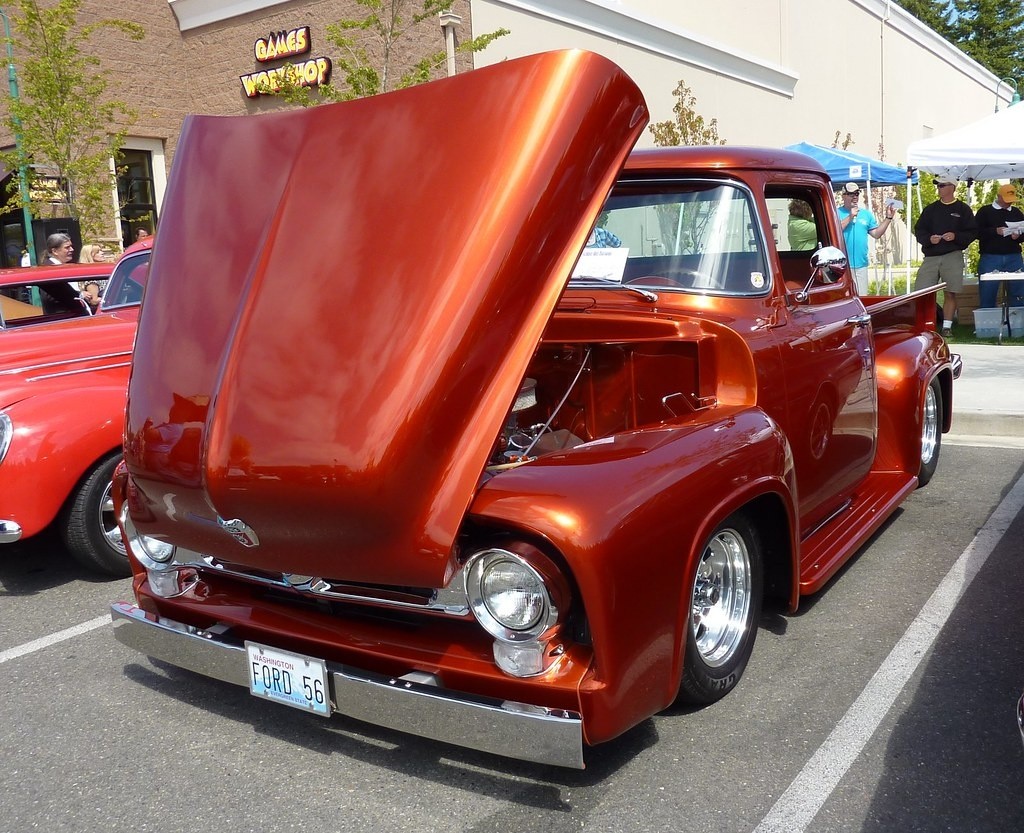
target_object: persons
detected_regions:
[914,173,975,337]
[788,198,817,252]
[78,245,109,316]
[973,184,1024,311]
[133,228,148,240]
[587,225,621,248]
[837,181,898,295]
[36,232,93,315]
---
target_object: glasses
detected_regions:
[937,184,952,189]
[843,192,859,197]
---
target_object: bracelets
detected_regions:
[886,216,893,220]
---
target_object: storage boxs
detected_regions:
[973,307,1024,339]
[954,284,1005,326]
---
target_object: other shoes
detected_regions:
[940,328,954,337]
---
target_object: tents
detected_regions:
[780,140,919,295]
[908,99,1024,344]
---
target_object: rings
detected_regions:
[947,237,949,239]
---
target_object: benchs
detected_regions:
[617,248,816,296]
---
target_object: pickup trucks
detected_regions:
[110,42,962,771]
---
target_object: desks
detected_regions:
[980,271,1024,345]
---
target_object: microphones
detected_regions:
[852,204,858,224]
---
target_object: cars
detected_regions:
[0,236,158,578]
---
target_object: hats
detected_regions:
[933,174,958,187]
[841,182,862,194]
[998,184,1018,203]
